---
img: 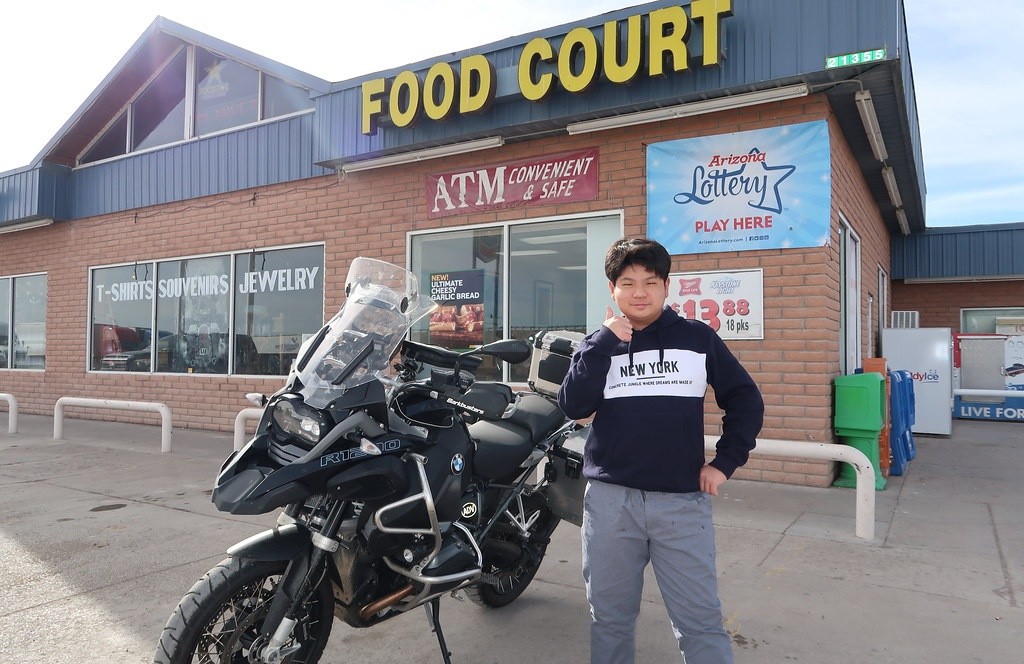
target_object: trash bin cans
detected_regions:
[835,368,914,492]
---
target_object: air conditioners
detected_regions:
[881,167,902,208]
[891,310,920,329]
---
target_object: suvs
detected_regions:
[99,331,260,375]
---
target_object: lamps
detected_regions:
[0,218,53,234]
[567,84,811,135]
[855,90,888,163]
[897,208,912,235]
[341,136,505,173]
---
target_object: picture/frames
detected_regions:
[534,278,553,328]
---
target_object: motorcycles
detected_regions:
[150,257,593,664]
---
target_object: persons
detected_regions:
[558,238,765,664]
